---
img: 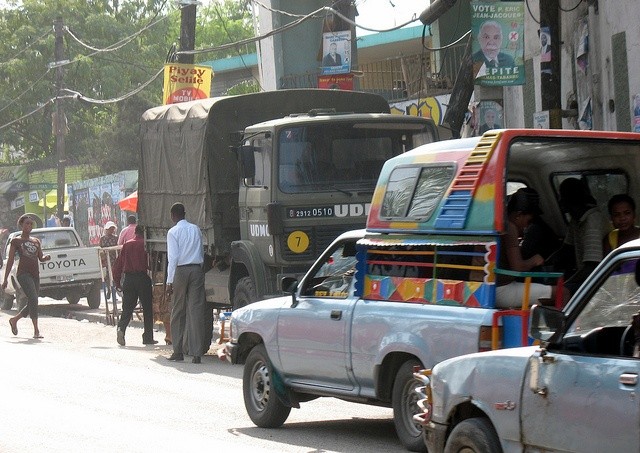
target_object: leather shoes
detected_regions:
[166,354,183,361]
[192,356,201,363]
[117,328,125,345]
[143,341,158,343]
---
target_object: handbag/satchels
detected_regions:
[159,291,172,323]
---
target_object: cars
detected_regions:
[413,237,638,449]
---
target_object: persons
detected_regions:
[495,177,640,311]
[165,202,208,363]
[323,43,342,66]
[100,221,120,298]
[117,216,138,244]
[2,216,51,338]
[112,226,158,346]
[539,34,551,54]
[474,22,515,68]
[480,110,501,136]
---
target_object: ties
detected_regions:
[491,59,495,67]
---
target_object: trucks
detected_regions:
[135,87,441,340]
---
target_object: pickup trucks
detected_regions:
[226,127,639,453]
[2,228,102,311]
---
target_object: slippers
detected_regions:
[9,319,17,335]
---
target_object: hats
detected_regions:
[104,221,117,230]
